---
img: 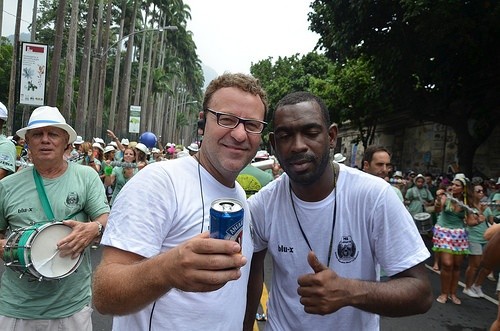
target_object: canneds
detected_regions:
[208,198,244,255]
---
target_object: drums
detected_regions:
[3,221,85,282]
[413,212,435,235]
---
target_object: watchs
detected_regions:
[95,220,104,237]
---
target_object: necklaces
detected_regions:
[287,164,338,270]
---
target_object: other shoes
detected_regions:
[472,283,485,297]
[487,273,496,281]
[433,263,439,270]
[255,313,268,321]
[448,295,462,305]
[463,287,479,297]
[91,241,100,249]
[437,294,447,303]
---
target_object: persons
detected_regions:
[363,145,391,277]
[333,153,346,164]
[243,92,435,331]
[92,73,269,331]
[0,100,281,250]
[0,107,110,331]
[391,170,500,331]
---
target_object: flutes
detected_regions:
[441,192,480,216]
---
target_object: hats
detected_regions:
[91,137,176,155]
[452,173,470,184]
[414,174,426,184]
[332,153,346,163]
[496,177,500,184]
[16,106,77,144]
[72,136,84,144]
[253,150,269,159]
[188,143,199,152]
[392,170,403,178]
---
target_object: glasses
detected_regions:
[410,176,414,179]
[474,189,484,193]
[204,108,268,134]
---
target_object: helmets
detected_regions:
[0,102,8,121]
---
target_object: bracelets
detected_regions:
[435,204,441,207]
[275,175,280,177]
